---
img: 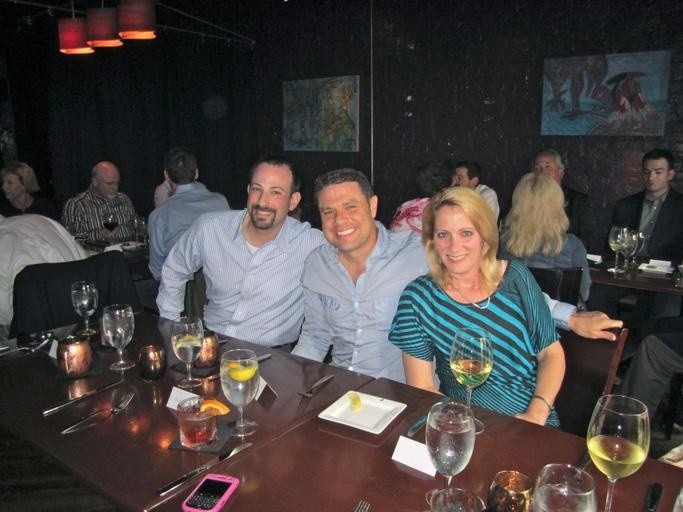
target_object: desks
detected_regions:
[1,313,373,511]
[589,254,682,299]
[80,236,155,282]
[150,377,683,512]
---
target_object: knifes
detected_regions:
[406,394,451,438]
[643,481,662,511]
[40,377,127,417]
[156,440,253,497]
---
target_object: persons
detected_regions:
[156,149,565,427]
[387,186,566,430]
[449,162,500,226]
[613,329,683,455]
[588,150,682,348]
[1,158,86,326]
[388,176,441,238]
[291,167,623,391]
[147,151,230,280]
[59,160,148,246]
[158,155,330,355]
[499,151,604,311]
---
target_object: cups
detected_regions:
[191,326,218,367]
[138,343,170,381]
[56,337,94,377]
[488,468,532,511]
[176,395,218,447]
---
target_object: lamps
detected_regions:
[55,1,96,57]
[84,1,125,49]
[116,2,157,41]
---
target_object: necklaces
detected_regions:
[451,277,490,309]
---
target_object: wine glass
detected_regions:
[171,315,205,390]
[128,215,146,243]
[100,211,119,240]
[449,325,494,434]
[218,347,262,439]
[100,302,138,373]
[606,224,646,273]
[530,462,598,512]
[70,278,99,336]
[424,399,486,512]
[585,393,654,511]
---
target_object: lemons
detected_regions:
[230,367,257,381]
[348,393,362,411]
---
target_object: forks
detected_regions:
[353,499,372,512]
[58,391,136,436]
[295,374,333,399]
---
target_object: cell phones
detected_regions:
[179,471,240,511]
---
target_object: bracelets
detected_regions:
[531,395,553,411]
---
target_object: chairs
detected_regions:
[528,266,582,310]
[554,323,626,442]
[10,251,136,336]
